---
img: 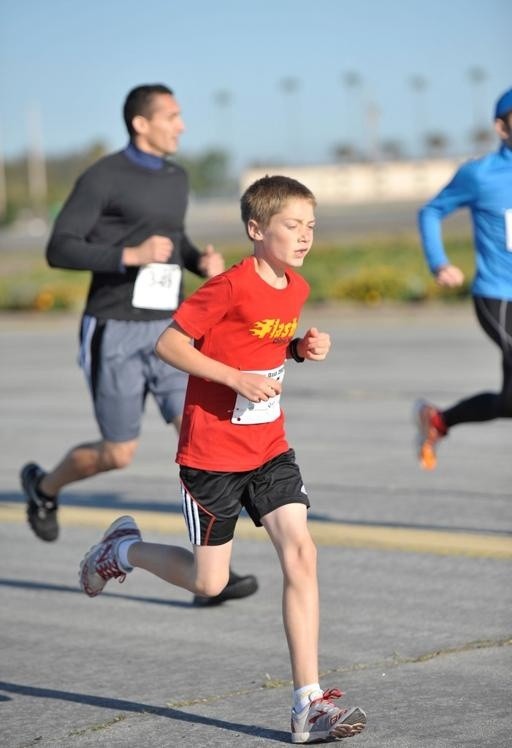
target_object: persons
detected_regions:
[80,175,368,744]
[416,90,511,476]
[20,83,258,607]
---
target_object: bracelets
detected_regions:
[288,336,303,363]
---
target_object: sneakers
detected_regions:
[79,516,140,597]
[22,464,58,541]
[291,688,366,743]
[419,404,447,470]
[194,569,257,605]
